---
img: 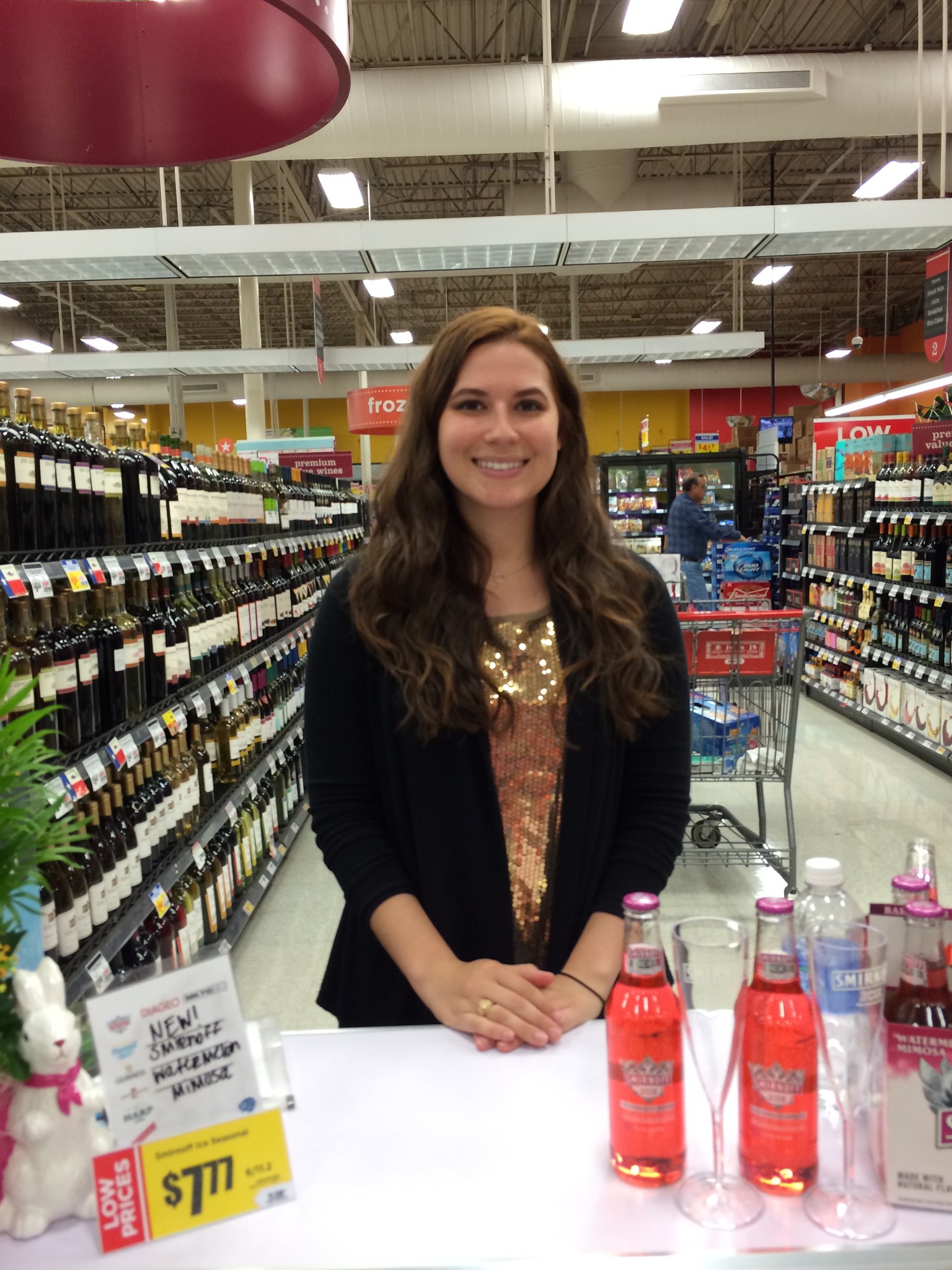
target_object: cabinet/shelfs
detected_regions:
[781,445,952,777]
[0,524,367,1011]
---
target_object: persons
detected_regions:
[303,305,693,1052]
[663,472,746,611]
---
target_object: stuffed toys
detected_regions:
[0,956,112,1239]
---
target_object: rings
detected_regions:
[477,999,494,1016]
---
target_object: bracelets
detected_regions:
[555,971,606,1005]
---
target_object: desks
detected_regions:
[0,1007,952,1270]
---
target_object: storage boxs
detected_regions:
[711,405,952,612]
[690,691,760,765]
[805,661,952,751]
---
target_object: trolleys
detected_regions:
[672,599,814,930]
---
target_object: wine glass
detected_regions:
[671,915,764,1231]
[802,920,896,1239]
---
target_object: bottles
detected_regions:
[843,671,854,682]
[875,452,896,507]
[809,582,876,621]
[805,618,852,642]
[882,900,952,1029]
[604,892,686,1188]
[171,635,305,973]
[0,571,166,780]
[37,743,178,1001]
[888,451,919,507]
[161,435,369,693]
[824,663,836,676]
[808,654,818,666]
[0,380,182,550]
[871,522,952,588]
[892,875,929,905]
[932,445,952,507]
[871,596,952,669]
[734,897,820,1196]
[906,839,938,904]
[917,454,940,507]
[793,857,867,1115]
[850,625,872,662]
